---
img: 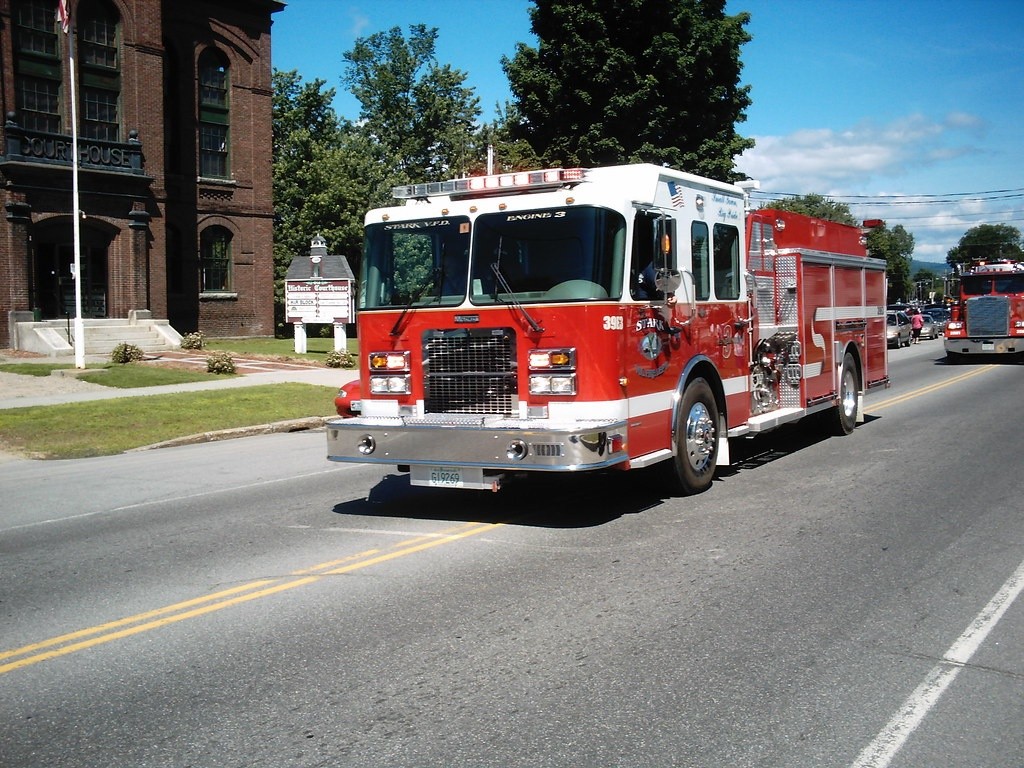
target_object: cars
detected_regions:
[888,299,951,341]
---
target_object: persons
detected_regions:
[911,309,924,344]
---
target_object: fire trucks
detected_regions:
[942,256,1024,360]
[326,141,891,497]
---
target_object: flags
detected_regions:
[56,0,71,36]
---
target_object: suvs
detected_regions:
[885,309,913,349]
